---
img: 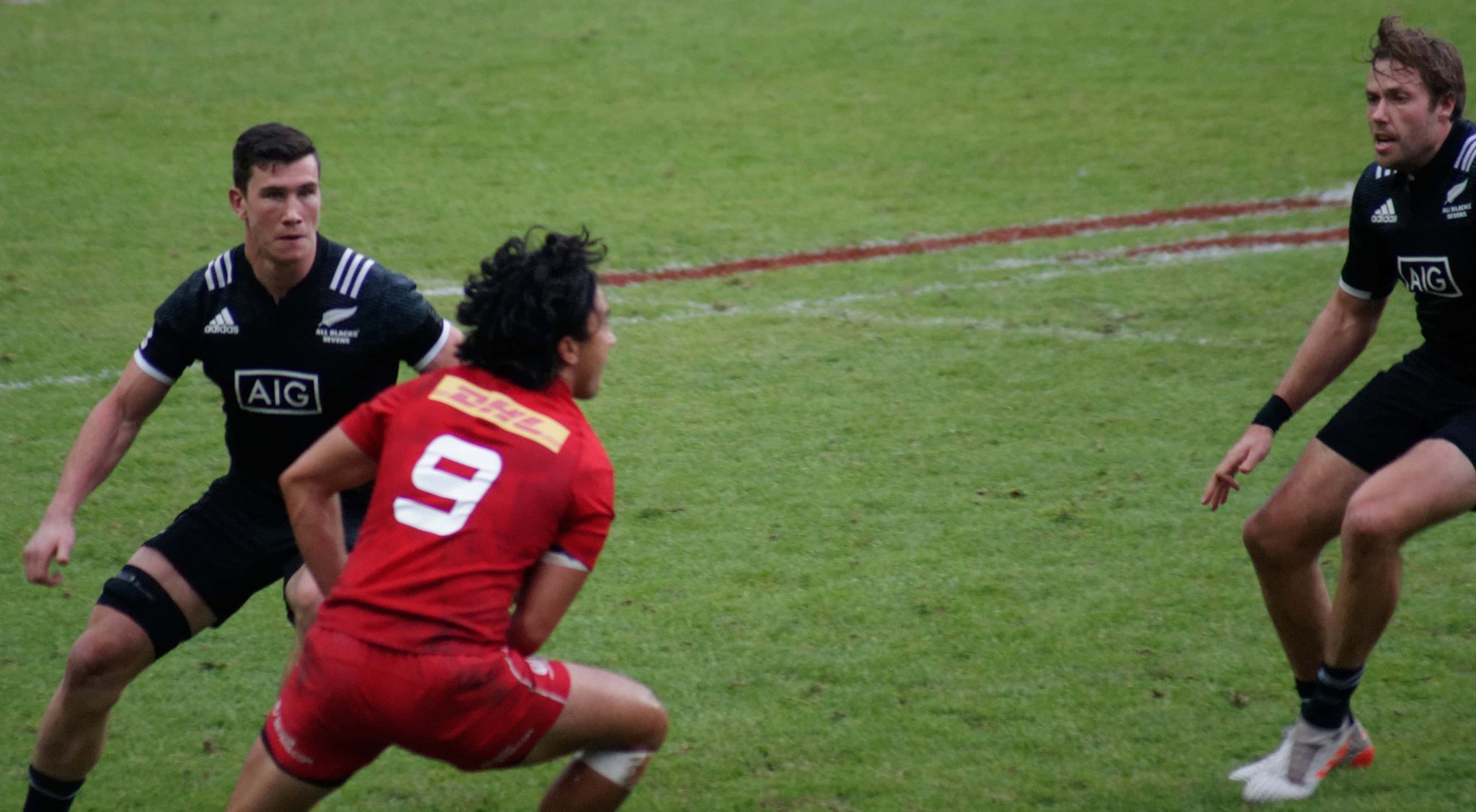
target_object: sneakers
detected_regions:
[1229,720,1377,781]
[1244,716,1356,803]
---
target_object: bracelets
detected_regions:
[1253,394,1291,434]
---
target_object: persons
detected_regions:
[1201,14,1476,800]
[24,122,466,812]
[224,225,667,812]
